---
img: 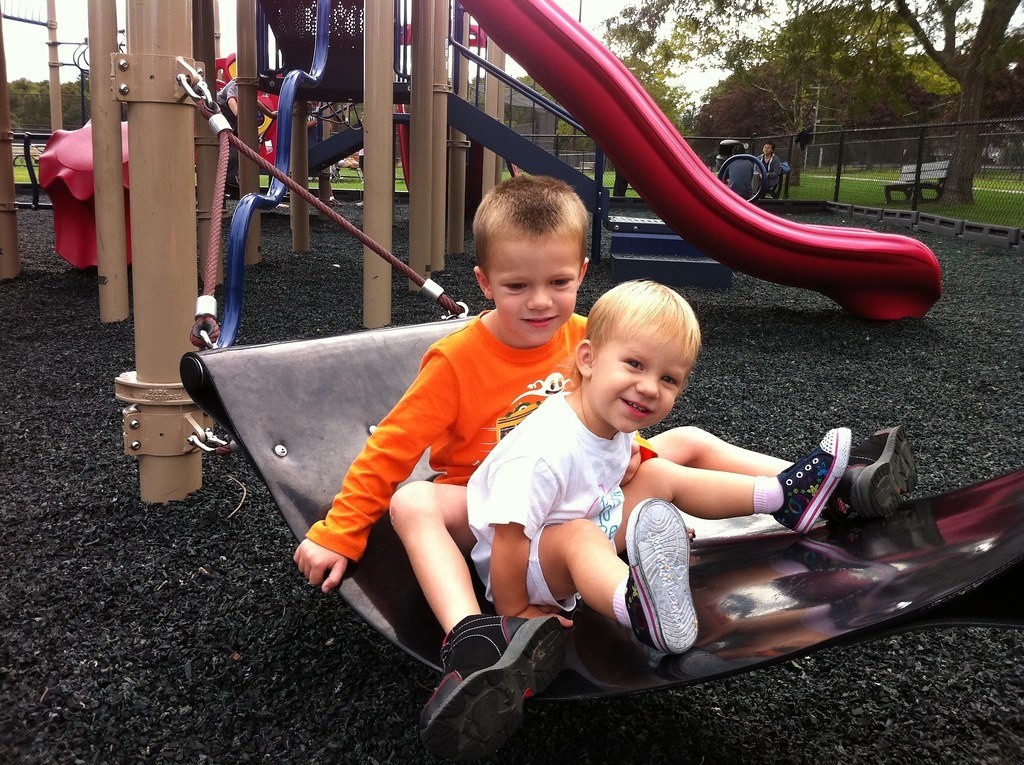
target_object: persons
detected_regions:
[216,78,240,186]
[465,279,853,652]
[750,142,780,199]
[291,175,921,765]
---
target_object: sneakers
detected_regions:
[820,424,920,526]
[418,614,567,763]
[768,427,853,535]
[623,498,698,654]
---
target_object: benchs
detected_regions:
[880,159,950,202]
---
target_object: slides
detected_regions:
[455,1,943,319]
